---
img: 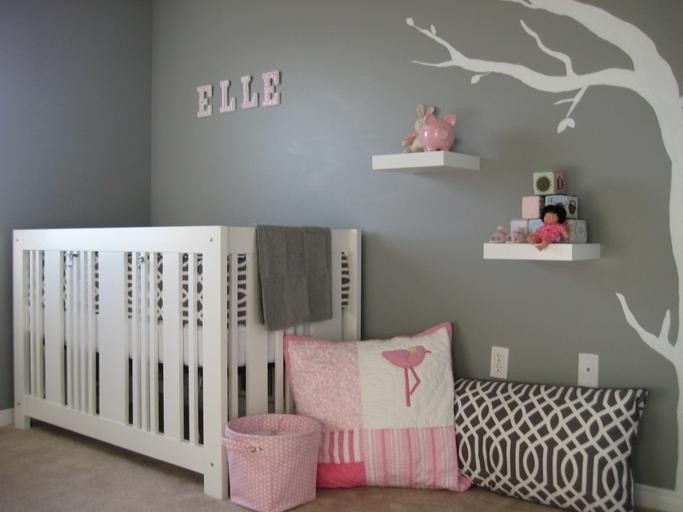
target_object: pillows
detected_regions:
[454,376,651,512]
[283,321,472,492]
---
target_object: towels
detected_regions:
[253,225,334,331]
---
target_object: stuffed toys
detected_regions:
[402,104,436,153]
[528,206,569,250]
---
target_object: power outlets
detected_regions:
[490,345,510,380]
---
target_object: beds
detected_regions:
[12,225,362,501]
